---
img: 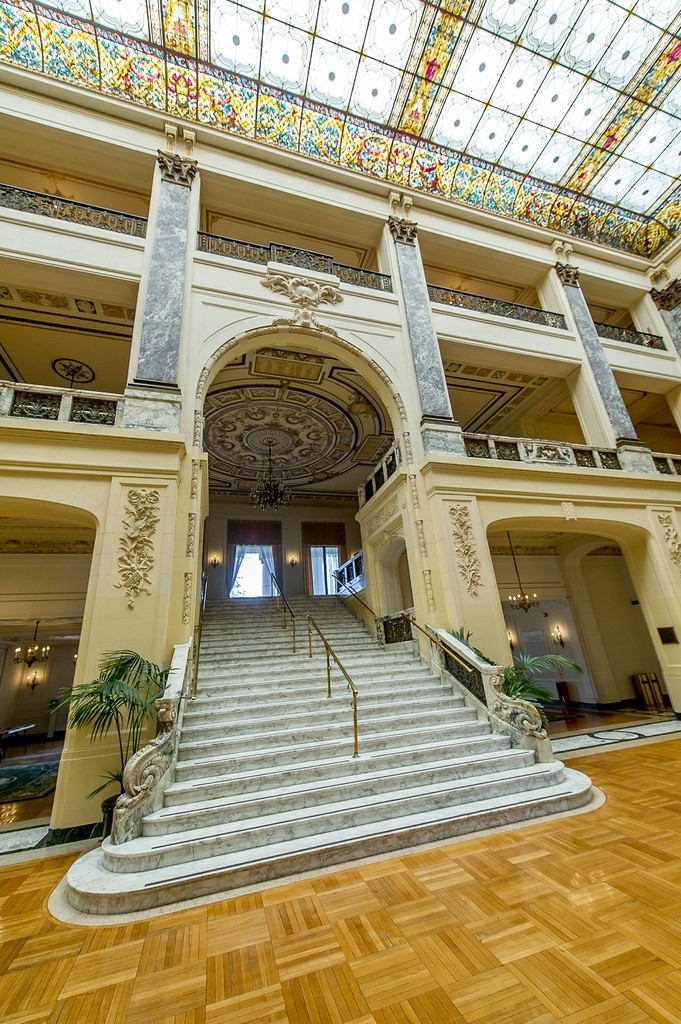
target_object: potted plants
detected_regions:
[47,649,178,838]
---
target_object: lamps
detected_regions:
[506,532,539,614]
[26,671,40,690]
[551,625,565,649]
[210,554,220,569]
[508,632,514,652]
[249,441,292,512]
[14,620,50,668]
[289,554,298,568]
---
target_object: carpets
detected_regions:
[0,760,60,805]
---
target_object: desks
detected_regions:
[0,723,36,763]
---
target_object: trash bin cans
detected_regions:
[633,672,664,706]
